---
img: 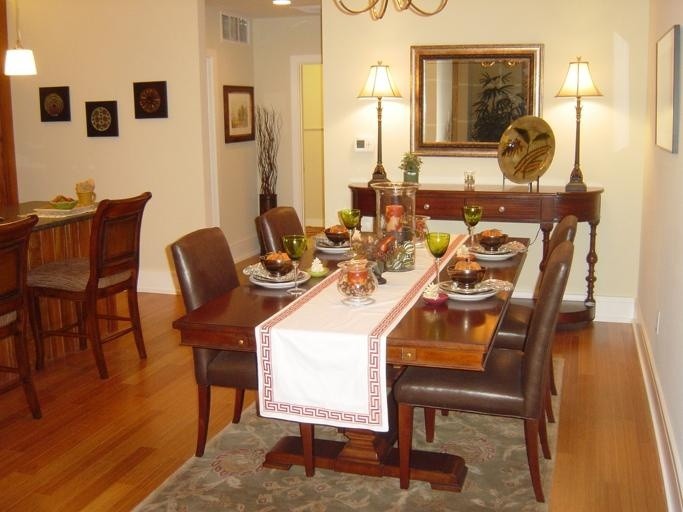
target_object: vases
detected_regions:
[258,193,277,255]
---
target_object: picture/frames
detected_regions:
[223,84,256,144]
[654,24,681,154]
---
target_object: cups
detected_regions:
[464,171,474,187]
[76,191,95,206]
[414,215,431,246]
[370,182,420,272]
[337,260,376,306]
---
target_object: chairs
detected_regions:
[165,227,347,478]
[254,205,305,255]
[27,190,153,381]
[1,214,44,421]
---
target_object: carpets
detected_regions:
[129,351,566,511]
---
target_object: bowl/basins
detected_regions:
[49,201,76,209]
[323,229,349,245]
[259,255,292,276]
[479,233,507,251]
[447,265,486,289]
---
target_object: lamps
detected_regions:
[3,0,39,77]
[555,55,606,193]
[356,59,403,188]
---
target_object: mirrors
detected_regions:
[409,41,545,158]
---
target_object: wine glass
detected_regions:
[426,232,449,296]
[282,235,307,294]
[463,206,483,248]
[341,209,360,239]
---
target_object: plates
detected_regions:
[248,271,311,289]
[317,240,350,247]
[307,267,329,277]
[473,252,515,261]
[421,293,446,304]
[498,116,555,183]
[440,281,494,294]
[314,246,349,254]
[473,245,510,254]
[439,288,498,301]
[33,208,70,215]
[253,270,305,283]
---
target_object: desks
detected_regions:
[348,182,605,329]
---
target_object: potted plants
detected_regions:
[398,152,424,185]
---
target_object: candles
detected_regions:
[385,204,404,234]
[347,268,368,286]
